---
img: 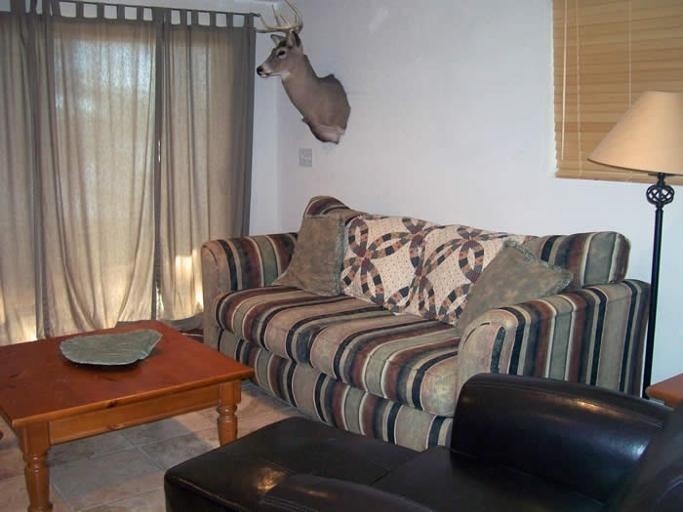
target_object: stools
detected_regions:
[164,408,417,512]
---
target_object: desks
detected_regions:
[0,317,257,512]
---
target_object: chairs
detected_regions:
[261,365,683,510]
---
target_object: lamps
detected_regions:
[587,86,682,401]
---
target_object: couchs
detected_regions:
[193,192,654,451]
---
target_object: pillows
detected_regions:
[456,240,573,337]
[401,225,504,326]
[273,212,347,296]
[338,213,436,313]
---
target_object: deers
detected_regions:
[251,1,351,146]
